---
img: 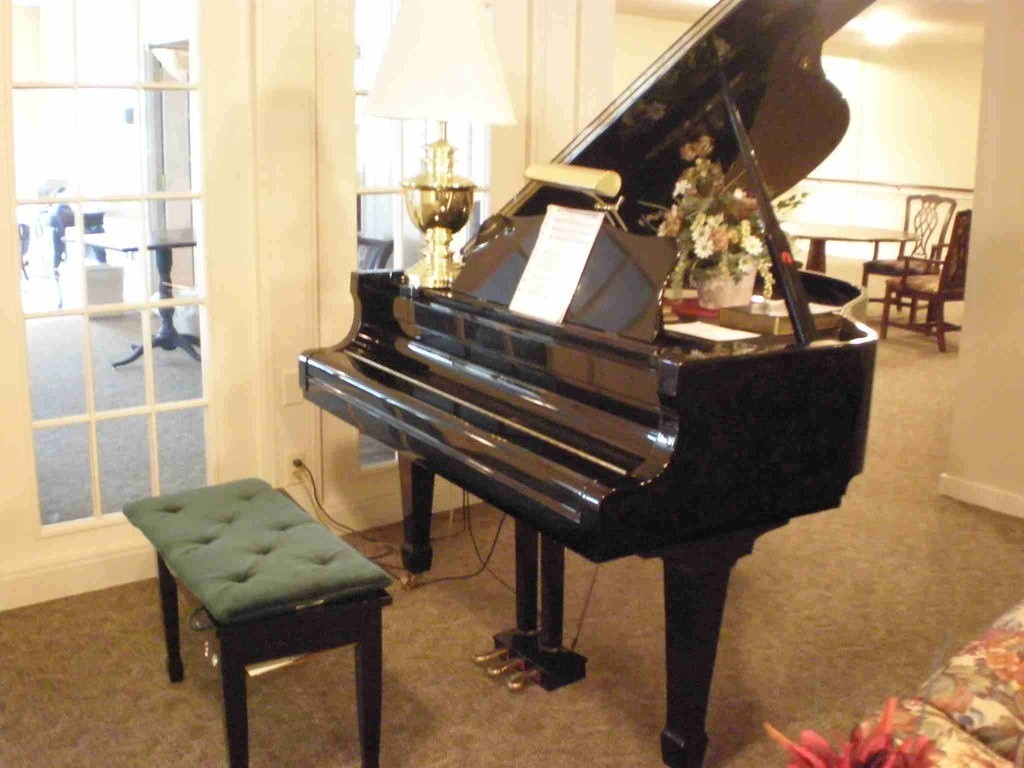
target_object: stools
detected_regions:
[123,477,392,765]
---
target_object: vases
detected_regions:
[692,261,759,310]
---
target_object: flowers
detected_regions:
[650,133,809,300]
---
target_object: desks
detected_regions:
[777,221,922,276]
[58,226,201,368]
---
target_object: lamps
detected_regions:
[365,0,516,289]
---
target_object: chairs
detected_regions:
[862,194,956,312]
[880,209,972,353]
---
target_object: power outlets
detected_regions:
[286,453,307,485]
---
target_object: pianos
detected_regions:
[293,0,882,766]
